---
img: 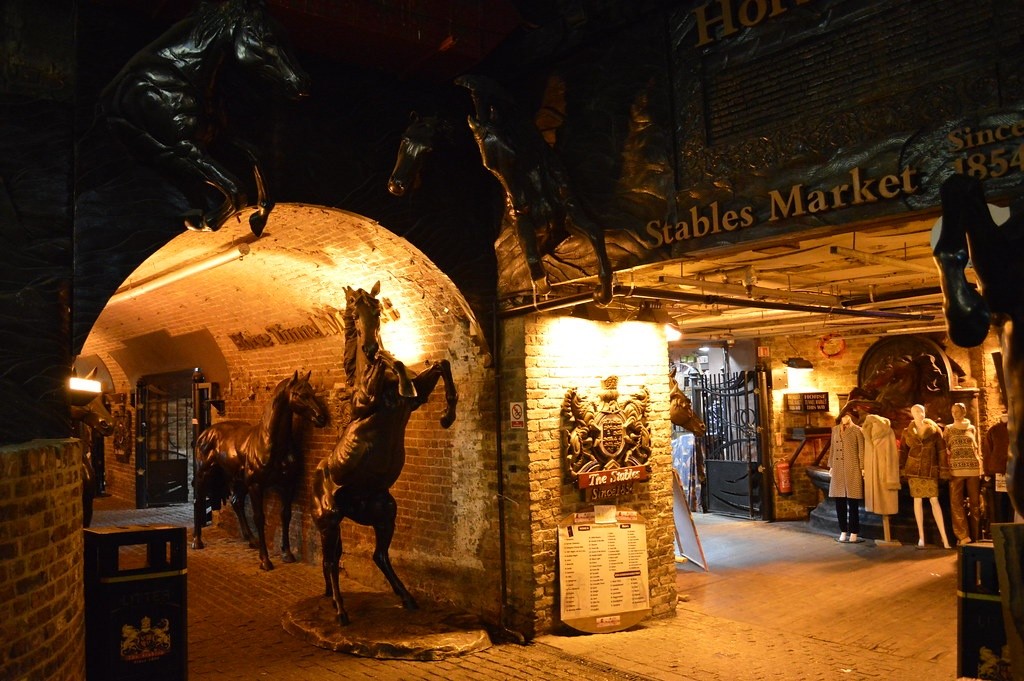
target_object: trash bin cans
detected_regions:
[83,521,189,681]
[955,539,1010,681]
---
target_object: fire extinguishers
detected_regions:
[771,455,792,496]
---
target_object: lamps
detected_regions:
[68,377,102,394]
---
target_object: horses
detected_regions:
[836,353,946,440]
[191,370,328,571]
[70,366,116,528]
[101,1,315,239]
[669,367,706,437]
[388,73,615,309]
[620,385,655,466]
[561,386,601,477]
[310,280,457,626]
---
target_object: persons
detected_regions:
[898,404,952,549]
[982,414,1011,539]
[826,415,865,543]
[943,403,983,547]
